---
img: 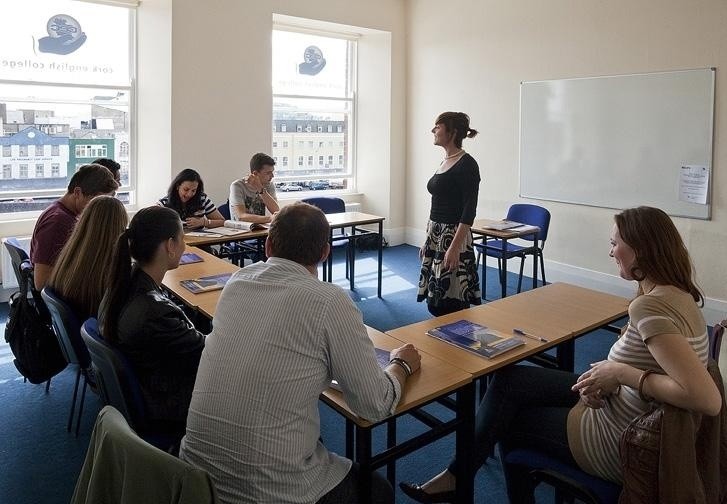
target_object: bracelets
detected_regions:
[204,218,211,227]
[386,357,421,378]
[637,368,657,404]
[259,187,268,194]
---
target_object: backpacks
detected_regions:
[5,275,68,392]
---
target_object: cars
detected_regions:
[308,181,327,190]
[279,183,303,193]
[12,197,34,203]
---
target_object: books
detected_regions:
[178,252,204,265]
[425,321,528,365]
[178,273,233,295]
[328,346,390,393]
[203,219,266,238]
[482,219,541,234]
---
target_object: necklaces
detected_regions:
[442,150,467,163]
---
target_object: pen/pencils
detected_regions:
[512,329,549,343]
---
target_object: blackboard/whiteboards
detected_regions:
[518,67,717,221]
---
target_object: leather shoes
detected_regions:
[400,481,455,504]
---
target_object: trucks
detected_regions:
[329,179,345,189]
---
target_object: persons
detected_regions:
[91,158,123,196]
[399,206,724,503]
[27,165,120,294]
[416,112,481,317]
[177,200,419,504]
[48,196,130,377]
[228,153,280,263]
[95,206,208,459]
[153,168,226,231]
